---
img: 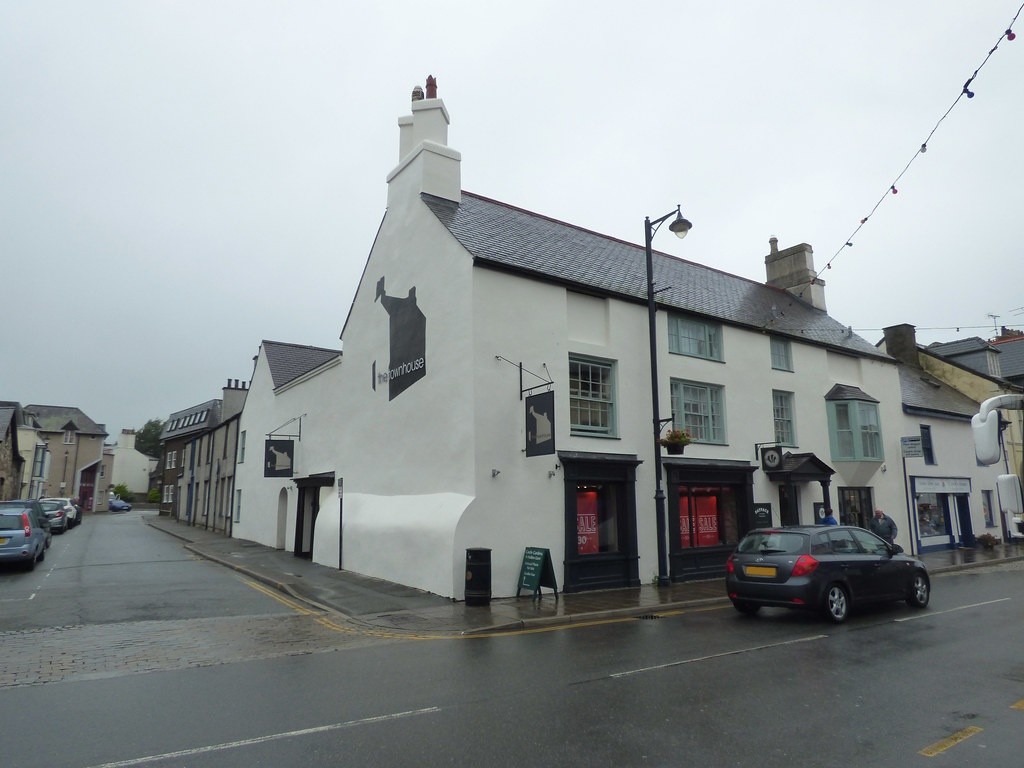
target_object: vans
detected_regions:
[109,491,120,500]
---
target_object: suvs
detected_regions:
[67,498,82,525]
[40,497,77,527]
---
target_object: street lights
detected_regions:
[643,204,692,585]
[60,449,69,498]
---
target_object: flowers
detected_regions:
[977,533,997,544]
[656,429,691,447]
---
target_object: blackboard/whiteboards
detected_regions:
[518,547,557,589]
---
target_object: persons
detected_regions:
[821,509,838,526]
[868,507,897,545]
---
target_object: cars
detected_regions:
[920,519,937,535]
[0,496,52,569]
[110,499,131,513]
[726,524,932,622]
[38,502,69,534]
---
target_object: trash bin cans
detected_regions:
[465,547,492,607]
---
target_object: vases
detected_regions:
[984,545,993,551]
[667,446,685,455]
